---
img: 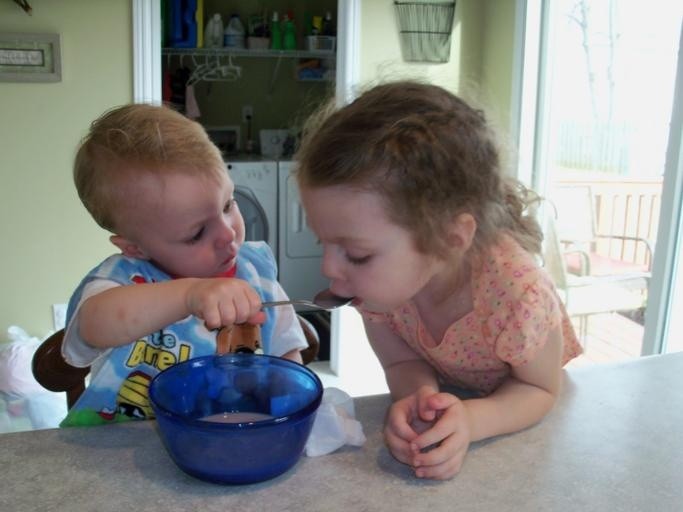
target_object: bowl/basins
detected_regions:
[146,352,322,484]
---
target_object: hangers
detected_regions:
[183,49,242,88]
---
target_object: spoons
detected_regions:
[260,287,354,311]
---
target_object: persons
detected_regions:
[297,78,585,486]
[55,102,309,432]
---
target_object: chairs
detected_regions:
[549,184,652,339]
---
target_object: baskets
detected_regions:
[305,35,335,52]
